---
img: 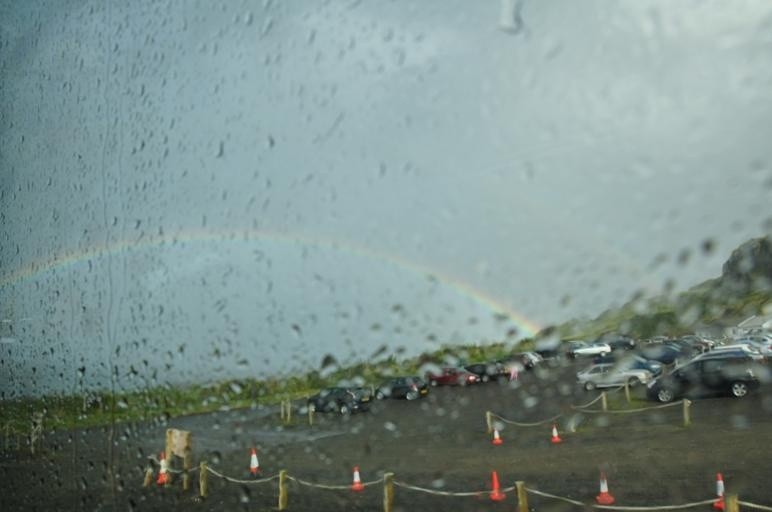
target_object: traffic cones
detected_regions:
[597,472,614,504]
[714,472,727,509]
[353,467,364,490]
[491,427,502,444]
[157,452,170,485]
[489,472,506,501]
[249,448,262,478]
[552,424,560,443]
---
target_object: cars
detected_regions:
[308,385,371,415]
[574,336,771,403]
[465,351,543,384]
[374,377,428,401]
[425,368,480,386]
